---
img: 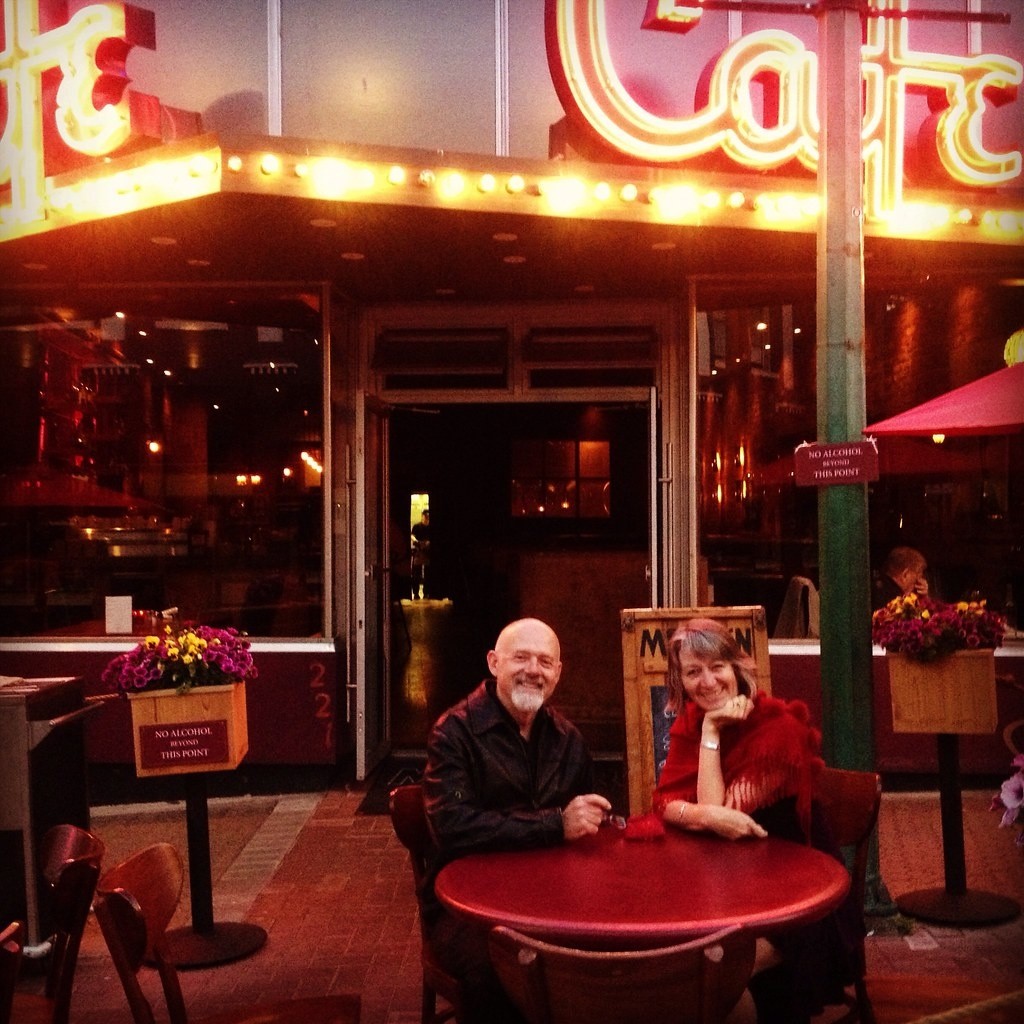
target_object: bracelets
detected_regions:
[701,740,721,751]
[677,802,688,820]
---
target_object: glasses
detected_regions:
[601,814,626,829]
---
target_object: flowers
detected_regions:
[990,753,1024,847]
[101,613,259,703]
[867,591,1004,654]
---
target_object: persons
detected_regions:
[419,617,626,1024]
[868,545,929,618]
[651,620,869,1023]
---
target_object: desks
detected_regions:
[433,820,850,1024]
[513,551,709,761]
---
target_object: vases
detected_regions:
[884,648,1001,733]
[129,681,246,775]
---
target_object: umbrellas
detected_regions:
[147,461,273,607]
[859,360,1023,447]
[1,462,174,616]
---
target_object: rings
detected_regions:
[737,702,741,708]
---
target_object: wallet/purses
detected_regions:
[624,815,665,839]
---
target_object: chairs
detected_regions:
[0,823,105,1024]
[487,924,757,1023]
[768,767,884,1024]
[389,782,481,1023]
[95,840,363,1023]
